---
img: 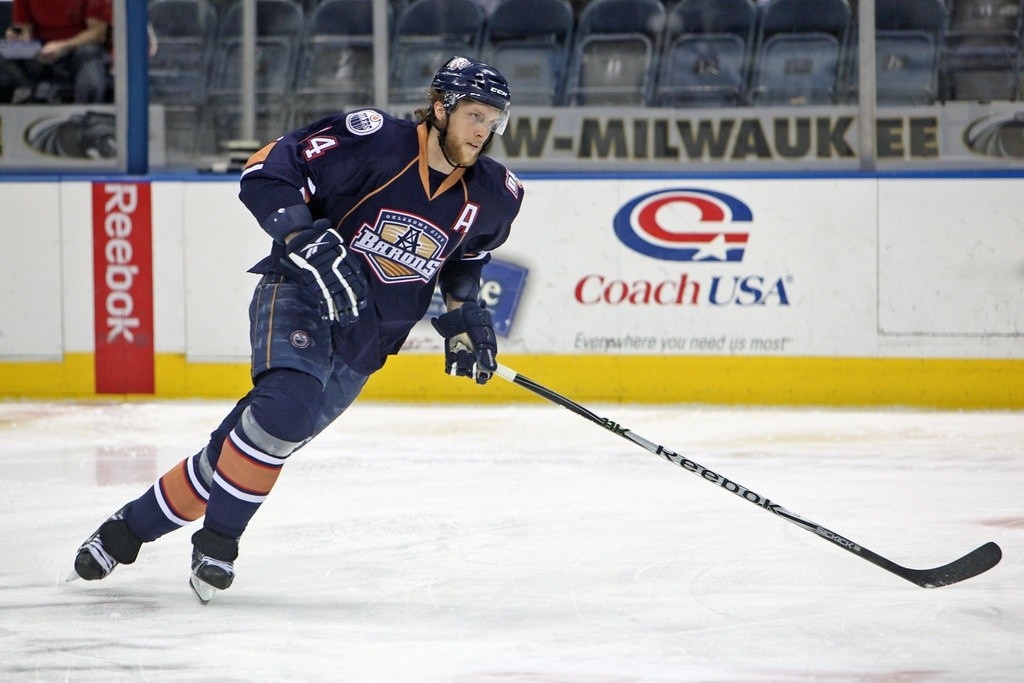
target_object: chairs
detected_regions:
[17,0,1024,156]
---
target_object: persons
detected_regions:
[65,54,524,605]
[0,0,114,104]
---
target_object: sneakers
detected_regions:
[65,501,144,583]
[190,528,240,605]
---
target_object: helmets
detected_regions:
[431,56,511,112]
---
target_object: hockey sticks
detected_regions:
[451,341,1004,590]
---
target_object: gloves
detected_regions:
[432,300,498,385]
[274,218,368,327]
